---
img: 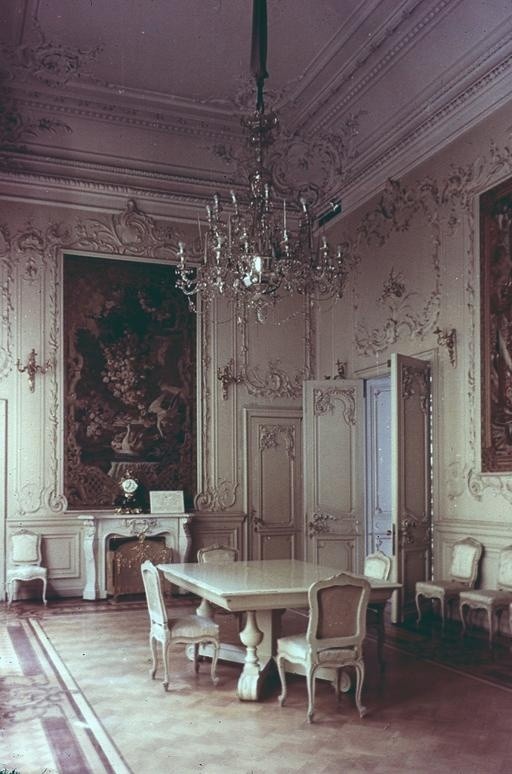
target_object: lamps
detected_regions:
[173,0,352,316]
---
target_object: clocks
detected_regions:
[118,469,142,513]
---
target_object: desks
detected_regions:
[155,558,402,701]
[78,514,193,601]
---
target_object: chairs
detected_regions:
[361,551,393,581]
[275,573,371,725]
[415,536,483,628]
[7,529,49,607]
[141,560,222,690]
[197,545,242,632]
[460,546,512,648]
